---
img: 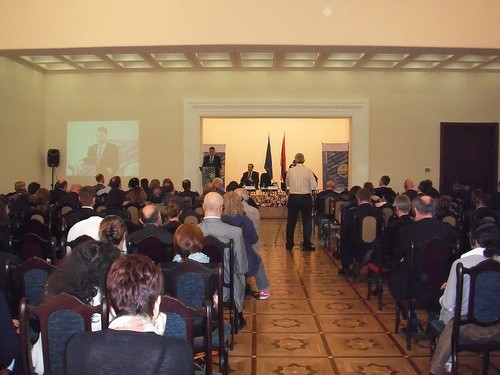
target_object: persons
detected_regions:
[66,206,128,256]
[202,146,222,178]
[337,189,375,281]
[364,182,374,194]
[173,224,221,370]
[199,192,248,330]
[0,286,38,375]
[404,174,500,243]
[100,215,129,254]
[428,218,499,375]
[162,182,186,210]
[127,205,173,245]
[65,253,196,375]
[229,181,239,192]
[285,153,317,253]
[388,194,455,338]
[221,192,271,301]
[163,196,184,232]
[314,180,339,242]
[373,175,395,196]
[0,196,9,229]
[8,174,173,211]
[368,194,412,297]
[212,178,226,194]
[206,183,216,193]
[87,127,119,175]
[66,186,98,231]
[338,186,361,226]
[180,179,199,198]
[243,164,259,185]
[234,187,261,254]
[45,240,123,331]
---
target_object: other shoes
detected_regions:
[372,287,384,296]
[354,265,361,276]
[400,316,419,333]
[338,266,351,275]
[427,313,438,332]
[304,245,317,251]
[286,243,293,249]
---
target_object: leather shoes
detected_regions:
[237,318,248,329]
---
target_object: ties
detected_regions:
[211,155,213,163]
[249,172,251,179]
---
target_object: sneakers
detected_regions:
[444,354,457,373]
[260,291,271,300]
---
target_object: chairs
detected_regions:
[1,190,500,375]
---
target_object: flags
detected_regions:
[264,136,273,179]
[280,135,286,180]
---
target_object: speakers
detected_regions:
[48,149,60,167]
